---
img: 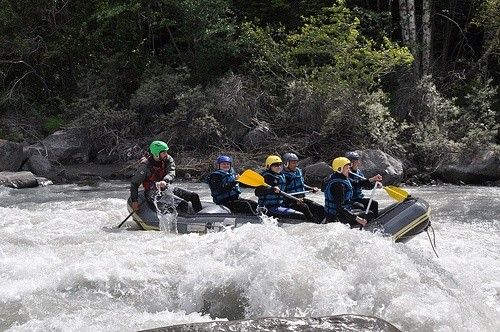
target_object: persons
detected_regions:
[324,157,383,227]
[130,141,203,214]
[281,153,325,222]
[205,156,258,216]
[255,155,322,224]
[330,151,382,218]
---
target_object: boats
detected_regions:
[127,189,433,245]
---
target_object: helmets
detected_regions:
[265,155,282,168]
[149,141,169,158]
[345,152,360,160]
[332,157,351,173]
[216,155,231,164]
[283,153,299,167]
